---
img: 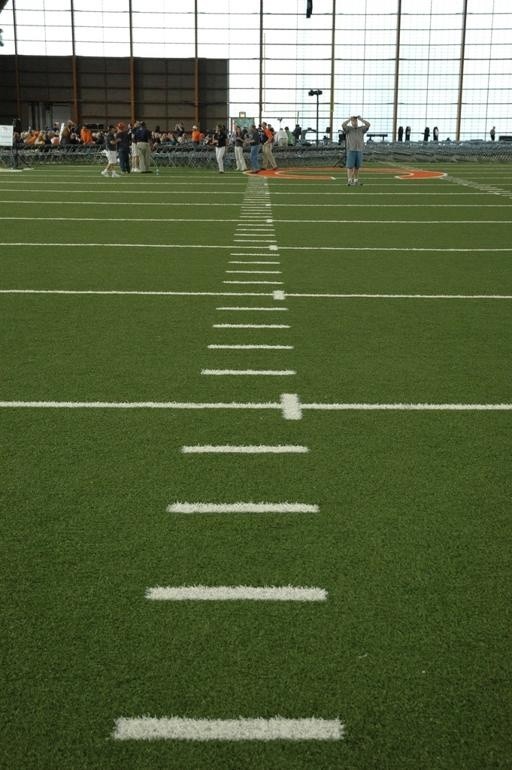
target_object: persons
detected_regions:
[490,127,495,141]
[323,135,328,146]
[342,115,370,188]
[13,116,312,178]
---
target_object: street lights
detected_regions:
[308,89,322,152]
